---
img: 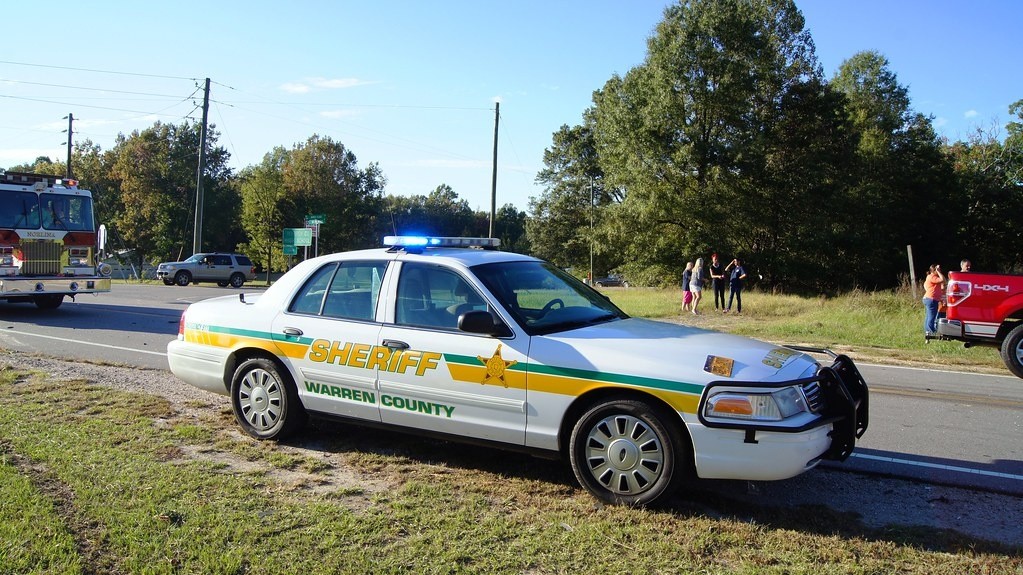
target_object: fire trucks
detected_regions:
[0,171,112,310]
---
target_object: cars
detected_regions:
[592,275,628,288]
[165,236,870,511]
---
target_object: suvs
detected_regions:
[156,251,255,288]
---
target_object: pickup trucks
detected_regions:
[925,270,1023,379]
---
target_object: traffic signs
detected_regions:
[305,214,326,225]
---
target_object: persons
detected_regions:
[934,284,946,331]
[710,253,726,313]
[689,258,709,315]
[960,260,971,273]
[44,200,64,223]
[922,264,946,340]
[682,262,694,311]
[725,258,746,314]
[254,261,262,273]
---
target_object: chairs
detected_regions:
[442,285,482,328]
[401,279,440,325]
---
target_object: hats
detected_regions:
[711,254,718,257]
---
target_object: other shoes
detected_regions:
[692,309,699,316]
[737,310,741,315]
[723,309,729,313]
